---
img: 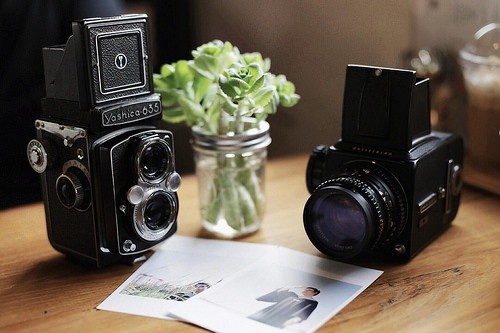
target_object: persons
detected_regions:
[165,283,211,302]
[244,283,321,328]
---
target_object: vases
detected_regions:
[190,118,274,239]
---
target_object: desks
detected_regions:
[1,151,500,333]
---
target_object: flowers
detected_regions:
[153,39,301,230]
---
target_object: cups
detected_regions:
[459,20,500,178]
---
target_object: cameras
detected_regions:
[26,11,182,276]
[302,65,466,269]
